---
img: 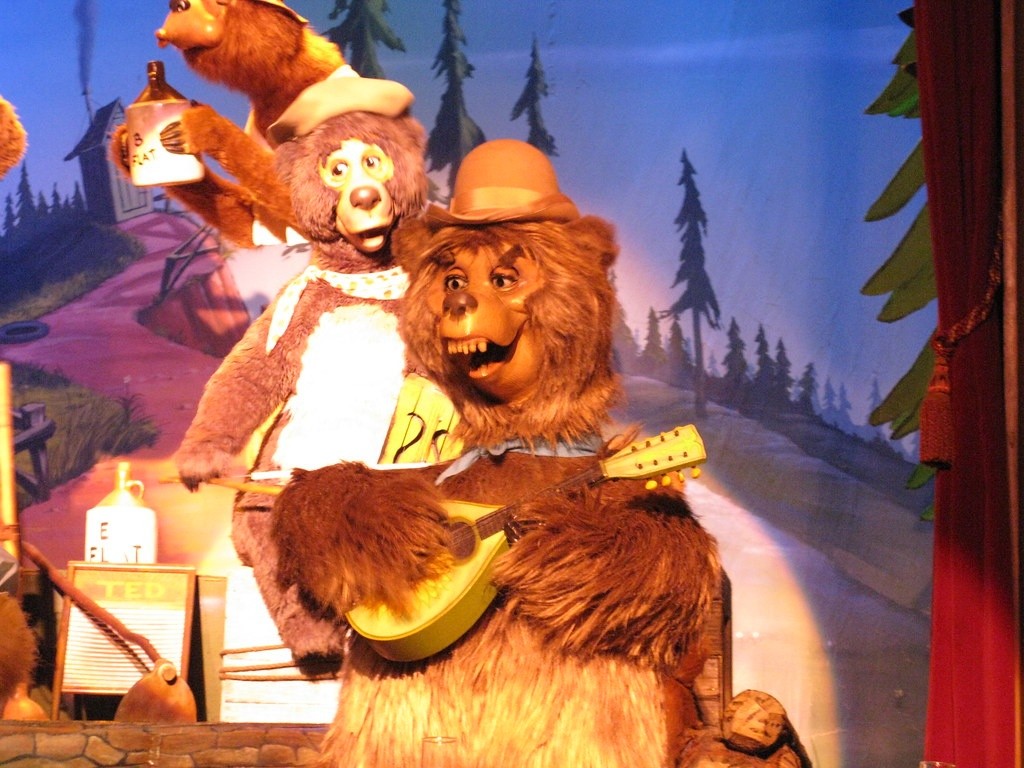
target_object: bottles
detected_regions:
[85,462,158,566]
[124,60,205,190]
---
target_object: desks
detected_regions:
[50,561,227,722]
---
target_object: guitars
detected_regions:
[342,422,708,662]
